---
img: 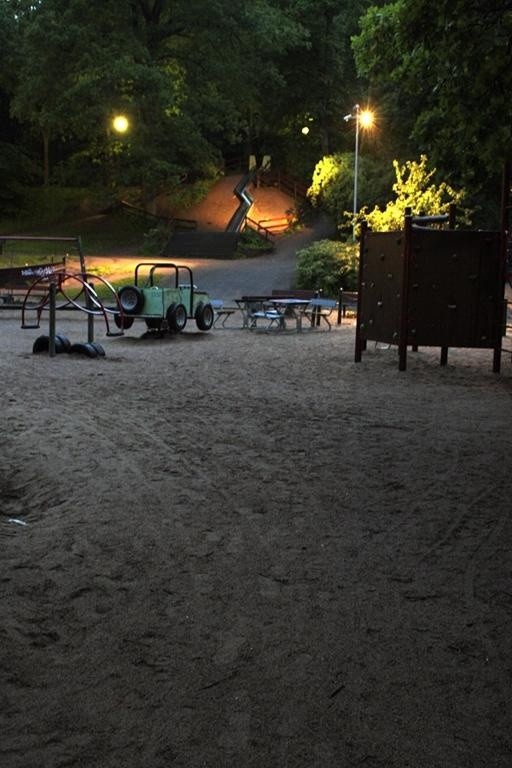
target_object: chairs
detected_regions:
[210,289,337,336]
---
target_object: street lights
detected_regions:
[344,100,378,243]
[301,110,320,164]
[100,104,134,219]
